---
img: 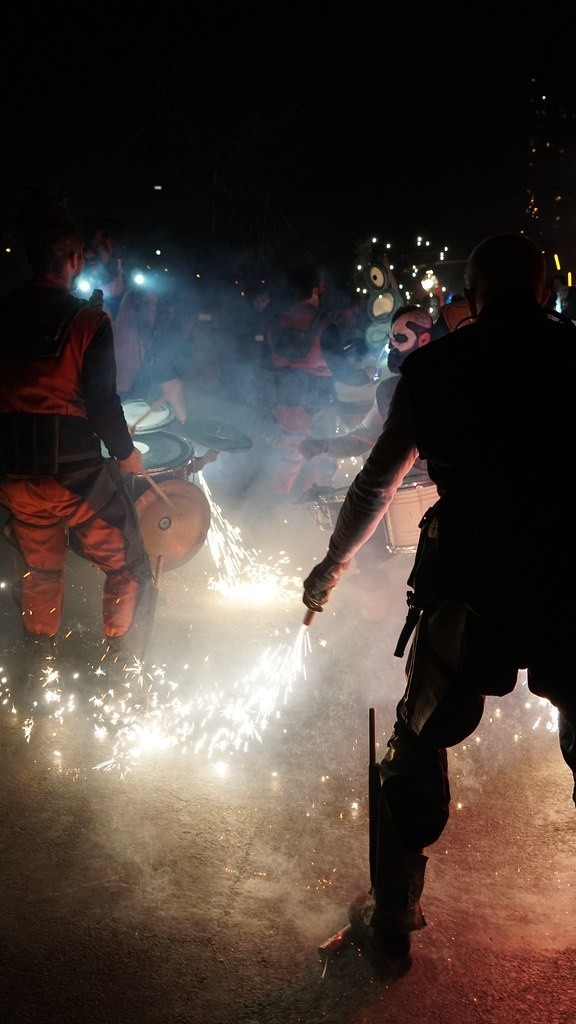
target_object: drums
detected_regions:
[102,430,195,491]
[317,472,440,555]
[119,399,179,434]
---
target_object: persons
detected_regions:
[303,233,576,980]
[0,223,576,511]
[0,203,154,714]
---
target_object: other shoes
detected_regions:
[349,893,410,973]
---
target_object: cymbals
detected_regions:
[133,480,213,574]
[181,418,254,454]
[299,369,374,436]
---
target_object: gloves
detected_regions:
[299,437,328,460]
[302,552,350,612]
